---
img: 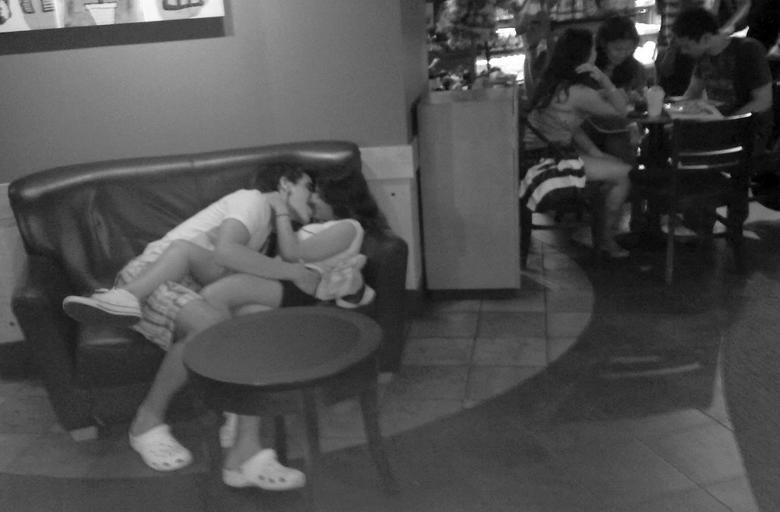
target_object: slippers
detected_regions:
[222,448,307,491]
[128,424,194,472]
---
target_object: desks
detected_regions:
[613,96,724,253]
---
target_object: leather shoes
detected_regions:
[605,247,630,258]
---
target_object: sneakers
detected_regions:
[63,289,142,328]
[219,411,238,449]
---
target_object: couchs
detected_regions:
[8,140,409,433]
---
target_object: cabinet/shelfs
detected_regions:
[414,82,523,299]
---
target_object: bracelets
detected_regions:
[273,212,292,219]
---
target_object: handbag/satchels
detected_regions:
[518,152,587,213]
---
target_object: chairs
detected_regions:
[635,111,757,288]
[516,112,617,272]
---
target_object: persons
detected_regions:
[671,7,772,257]
[109,158,321,492]
[514,26,636,261]
[56,164,387,448]
[654,1,752,98]
[717,1,780,62]
[580,16,649,235]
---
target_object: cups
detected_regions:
[645,84,667,117]
